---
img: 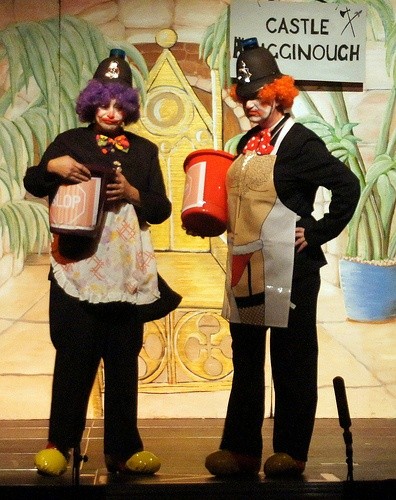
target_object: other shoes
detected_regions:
[205,450,262,480]
[116,451,161,476]
[263,452,306,480]
[34,448,68,476]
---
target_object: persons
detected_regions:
[24,58,179,477]
[182,49,361,484]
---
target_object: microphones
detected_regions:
[333,376,352,428]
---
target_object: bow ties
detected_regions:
[242,128,274,155]
[95,134,130,155]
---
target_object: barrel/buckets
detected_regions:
[181,150,235,236]
[48,164,111,239]
[339,256,396,324]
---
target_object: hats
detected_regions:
[236,37,283,97]
[93,49,132,89]
